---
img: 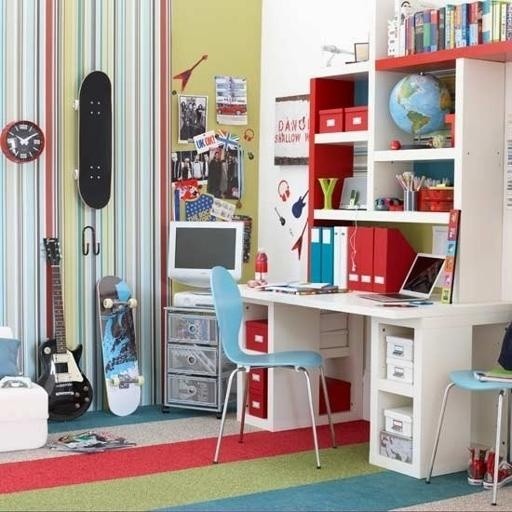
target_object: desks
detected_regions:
[236,0,512,480]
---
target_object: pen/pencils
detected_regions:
[379,301,433,307]
[396,172,450,192]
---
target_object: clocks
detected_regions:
[0,120,45,166]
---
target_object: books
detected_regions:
[472,368,512,383]
[261,284,339,295]
[257,279,331,290]
[398,0,512,55]
[46,428,137,453]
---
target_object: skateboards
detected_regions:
[72,71,112,209]
[96,275,144,415]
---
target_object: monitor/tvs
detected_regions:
[166,221,245,294]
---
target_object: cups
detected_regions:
[403,190,416,212]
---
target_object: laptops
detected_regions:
[358,252,446,303]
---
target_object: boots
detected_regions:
[482,449,512,490]
[466,446,486,486]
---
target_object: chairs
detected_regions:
[209,265,339,466]
[426,322,512,504]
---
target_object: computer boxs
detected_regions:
[173,291,215,309]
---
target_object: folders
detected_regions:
[310,226,333,287]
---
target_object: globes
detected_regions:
[389,72,452,149]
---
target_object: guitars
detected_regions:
[35,239,92,422]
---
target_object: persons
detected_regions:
[206,151,226,195]
[181,101,204,134]
[172,150,209,181]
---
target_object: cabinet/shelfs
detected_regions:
[162,306,237,419]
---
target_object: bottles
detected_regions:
[254,248,269,283]
